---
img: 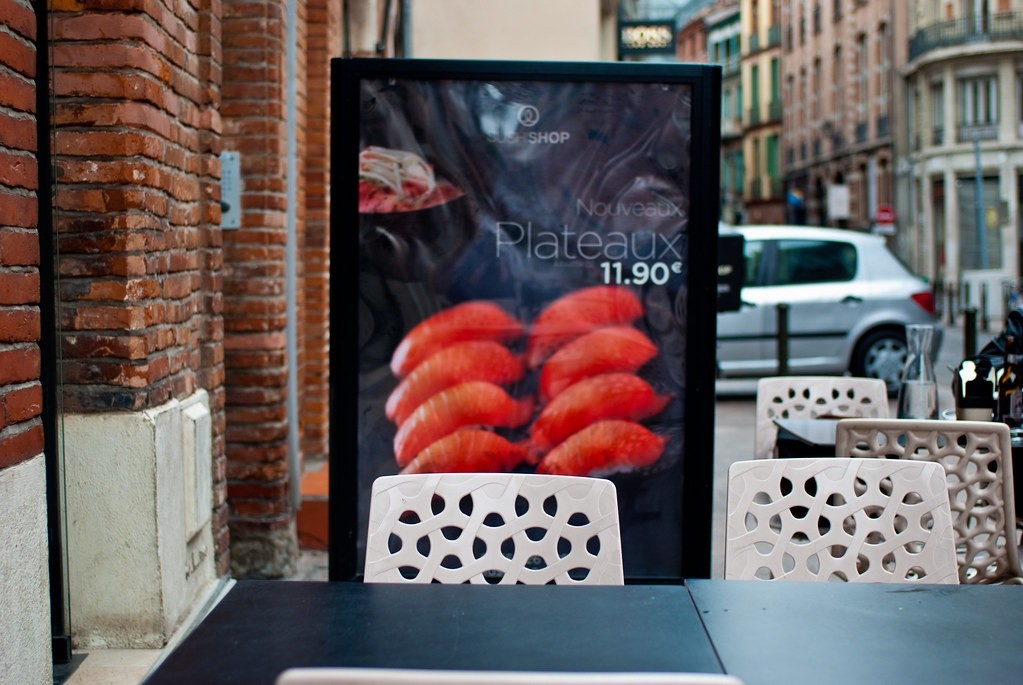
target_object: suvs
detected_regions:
[712,221,943,398]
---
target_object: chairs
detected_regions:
[723,457,961,590]
[753,376,891,462]
[360,471,627,587]
[830,418,1023,586]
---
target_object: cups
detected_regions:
[892,321,942,422]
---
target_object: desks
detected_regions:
[134,580,729,682]
[681,577,1023,685]
[770,418,1023,532]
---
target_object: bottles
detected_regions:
[950,301,994,448]
[998,336,1022,429]
